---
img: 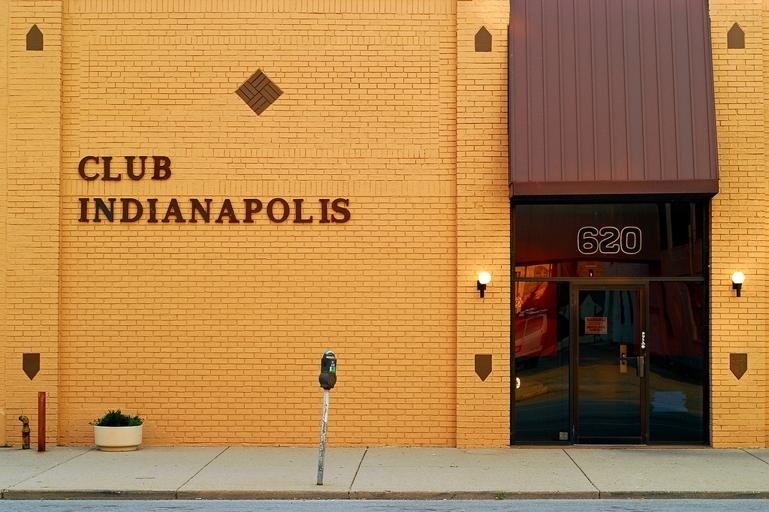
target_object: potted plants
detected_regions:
[89,408,145,452]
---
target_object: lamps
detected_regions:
[476,271,490,297]
[731,272,745,297]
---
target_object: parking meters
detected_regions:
[314,349,339,487]
